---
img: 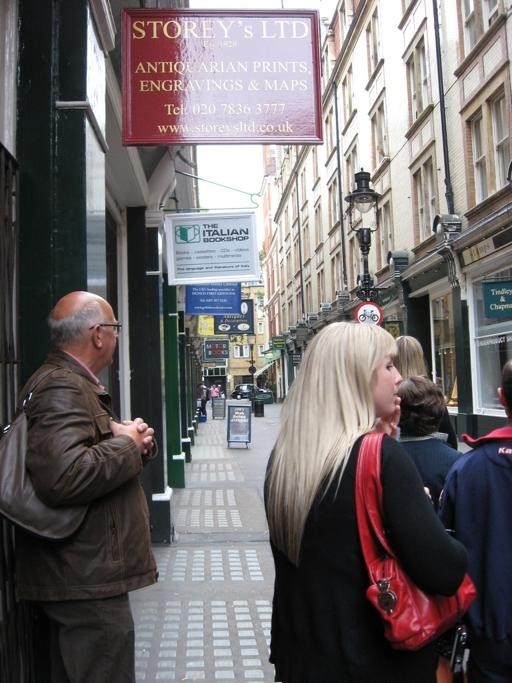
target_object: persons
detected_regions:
[14,291,161,683]
[197,383,225,415]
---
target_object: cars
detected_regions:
[231,384,270,401]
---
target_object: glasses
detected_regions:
[89,321,123,331]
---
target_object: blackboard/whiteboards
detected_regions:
[228,405,252,442]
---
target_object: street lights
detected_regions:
[340,167,386,325]
[244,331,258,412]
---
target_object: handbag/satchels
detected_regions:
[0,367,89,540]
[355,431,478,650]
[431,624,469,683]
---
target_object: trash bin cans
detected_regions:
[252,396,264,416]
